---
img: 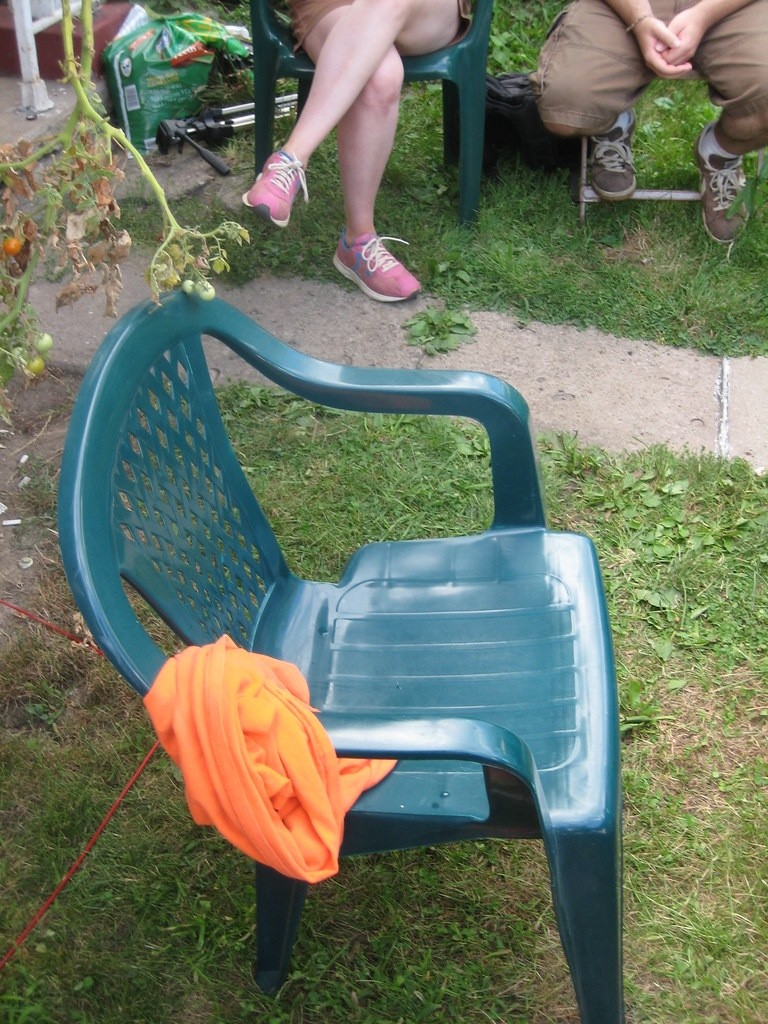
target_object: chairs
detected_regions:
[249,0,495,229]
[56,284,626,1024]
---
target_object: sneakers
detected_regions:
[332,229,421,302]
[694,122,748,244]
[591,107,637,202]
[241,150,309,228]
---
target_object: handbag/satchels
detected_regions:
[483,70,594,180]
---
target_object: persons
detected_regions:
[242,0,474,301]
[529,0,768,243]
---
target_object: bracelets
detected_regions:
[626,15,651,32]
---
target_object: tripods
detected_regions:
[153,94,297,175]
